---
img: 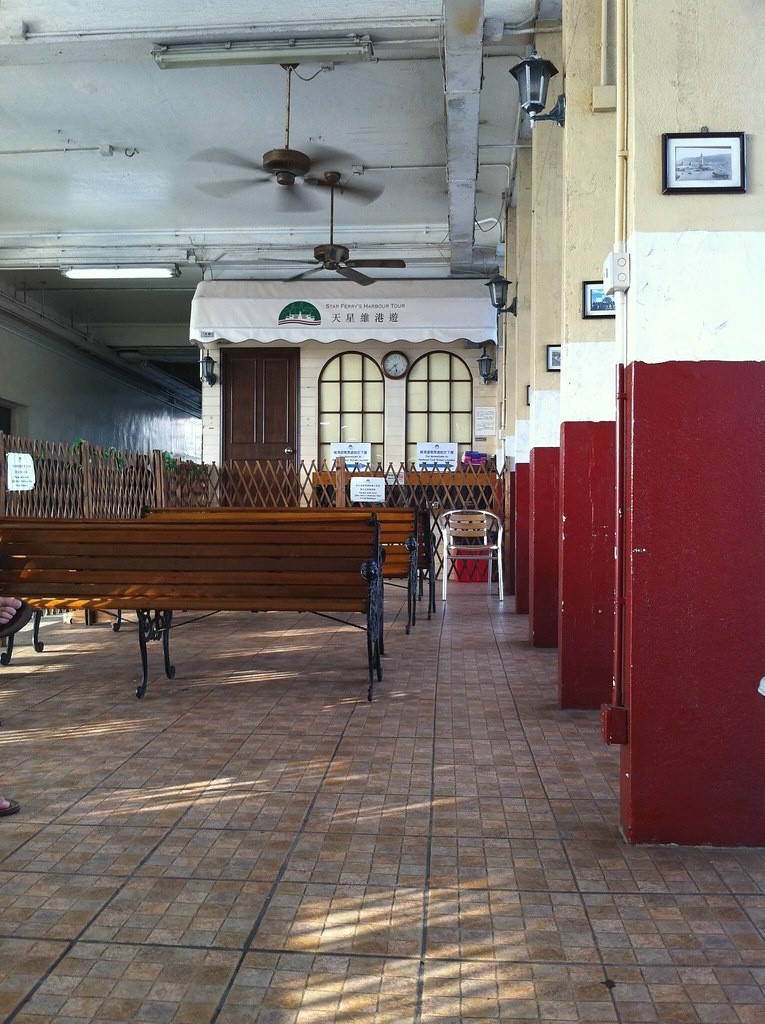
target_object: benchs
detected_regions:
[0,506,420,666]
[0,513,387,703]
[142,505,437,622]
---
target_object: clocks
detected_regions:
[380,351,411,380]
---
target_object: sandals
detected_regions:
[0,798,20,817]
[0,600,32,638]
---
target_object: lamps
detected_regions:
[199,349,217,388]
[509,43,565,127]
[151,34,379,71]
[59,264,182,278]
[476,346,499,383]
[484,265,517,317]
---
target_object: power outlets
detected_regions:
[321,62,335,71]
[351,165,364,175]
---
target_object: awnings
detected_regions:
[189,278,498,344]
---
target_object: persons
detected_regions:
[553,353,561,366]
[0,794,21,817]
[0,595,33,638]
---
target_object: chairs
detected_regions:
[439,509,504,601]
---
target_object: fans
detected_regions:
[185,62,387,212]
[258,171,406,286]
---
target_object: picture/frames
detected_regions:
[546,344,561,372]
[662,132,746,194]
[582,281,616,319]
[527,385,530,405]
